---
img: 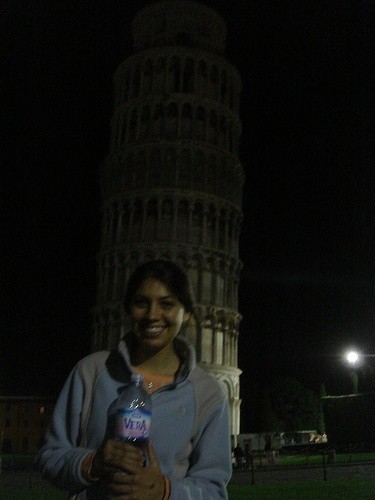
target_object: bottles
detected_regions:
[110,374,152,468]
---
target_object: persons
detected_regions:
[245,444,253,468]
[234,443,242,471]
[34,260,231,500]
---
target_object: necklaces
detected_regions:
[147,380,154,391]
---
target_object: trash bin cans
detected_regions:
[327,450,337,465]
